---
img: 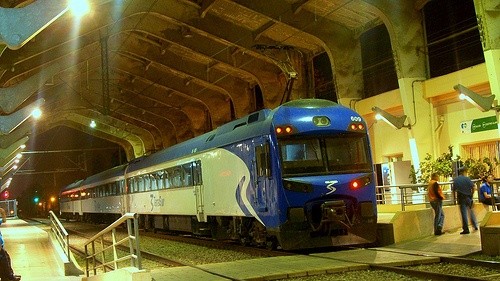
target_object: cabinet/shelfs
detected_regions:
[379,161,411,203]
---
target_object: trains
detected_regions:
[58,98,377,252]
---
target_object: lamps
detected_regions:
[371,106,414,130]
[0,0,84,185]
[454,84,500,113]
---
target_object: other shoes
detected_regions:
[0,274,22,281]
[460,231,470,234]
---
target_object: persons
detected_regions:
[0,207,21,281]
[428,173,446,236]
[452,168,478,234]
[480,174,500,211]
